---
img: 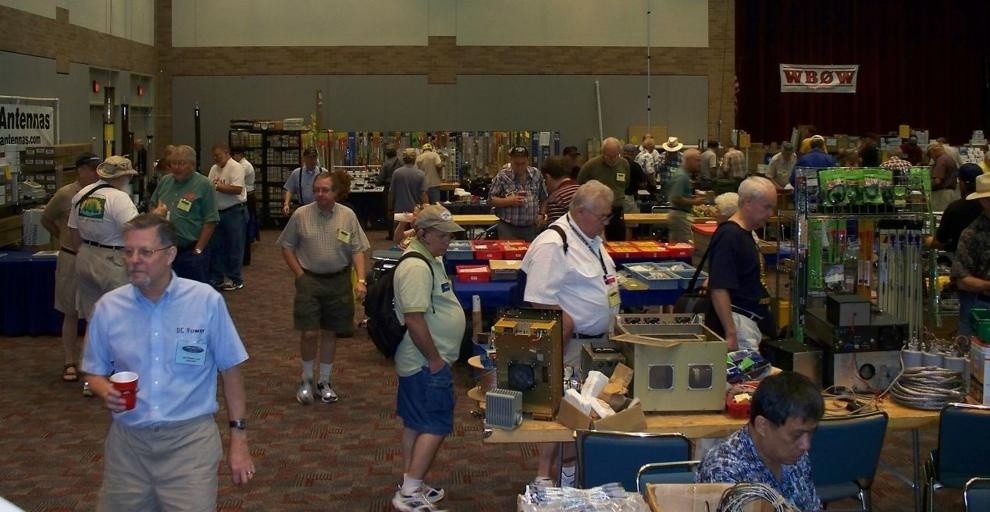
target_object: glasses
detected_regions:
[120,245,170,258]
[585,209,614,223]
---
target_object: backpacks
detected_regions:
[365,251,434,360]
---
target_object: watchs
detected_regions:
[228,418,248,431]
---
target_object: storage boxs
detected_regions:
[24,142,93,196]
[691,223,718,257]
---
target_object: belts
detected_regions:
[60,247,76,255]
[218,200,247,214]
[731,307,760,323]
[85,240,123,250]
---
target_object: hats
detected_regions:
[966,172,990,200]
[509,145,530,155]
[954,163,982,181]
[780,142,796,154]
[414,203,466,234]
[662,136,683,152]
[304,147,318,156]
[76,151,101,167]
[96,155,139,178]
[386,142,433,163]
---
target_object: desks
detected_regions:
[623,213,671,241]
[0,250,87,337]
[451,274,698,381]
[476,388,986,510]
[393,213,501,242]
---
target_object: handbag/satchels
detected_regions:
[674,286,722,336]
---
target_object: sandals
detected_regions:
[61,364,79,382]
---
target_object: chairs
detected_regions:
[809,411,892,510]
[636,459,705,494]
[570,426,693,492]
[920,400,989,511]
[960,476,989,511]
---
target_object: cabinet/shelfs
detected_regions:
[230,129,302,232]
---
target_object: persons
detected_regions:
[391,204,467,512]
[81,210,256,512]
[709,176,778,355]
[68,155,139,396]
[520,180,620,487]
[275,172,371,404]
[1,136,990,296]
[40,152,104,380]
[923,163,990,337]
[693,371,825,512]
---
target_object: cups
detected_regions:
[518,191,527,201]
[109,371,138,412]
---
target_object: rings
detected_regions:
[246,471,252,474]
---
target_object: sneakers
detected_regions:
[316,381,338,402]
[224,279,244,291]
[392,488,441,511]
[398,484,445,504]
[296,379,315,405]
